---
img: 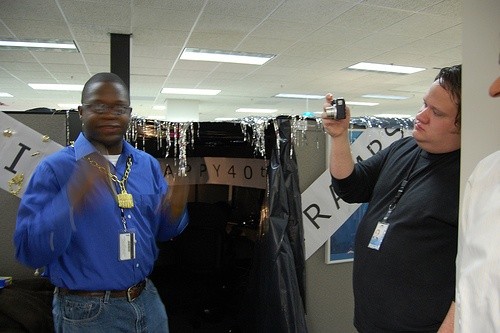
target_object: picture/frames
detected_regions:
[325,128,413,265]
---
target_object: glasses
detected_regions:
[82,102,131,115]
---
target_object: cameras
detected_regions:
[325,98,347,120]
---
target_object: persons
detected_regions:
[12,71,193,333]
[319,63,463,333]
[453,57,500,333]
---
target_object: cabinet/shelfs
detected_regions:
[0,110,415,333]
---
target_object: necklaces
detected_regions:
[84,152,138,211]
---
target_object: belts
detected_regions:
[54,279,148,302]
